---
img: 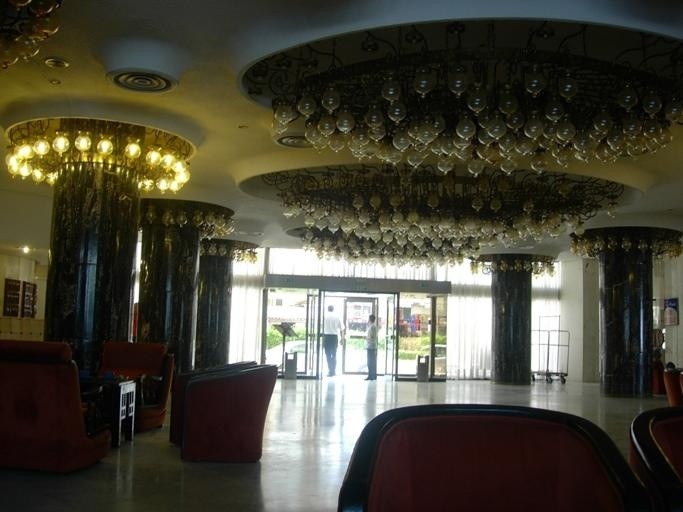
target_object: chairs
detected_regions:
[169,362,256,444]
[338,405,662,512]
[0,339,112,476]
[663,372,683,407]
[628,405,683,512]
[182,365,277,463]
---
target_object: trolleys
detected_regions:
[530,330,571,384]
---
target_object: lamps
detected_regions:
[569,227,683,263]
[5,119,196,195]
[301,228,481,268]
[238,15,683,177]
[198,239,259,264]
[470,254,556,279]
[140,198,237,238]
[269,162,629,250]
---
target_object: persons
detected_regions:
[363,315,379,380]
[320,306,345,377]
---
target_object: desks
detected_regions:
[86,378,137,447]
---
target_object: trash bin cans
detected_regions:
[417,355,430,382]
[428,317,438,335]
[284,352,297,379]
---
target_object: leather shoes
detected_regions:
[365,377,376,380]
